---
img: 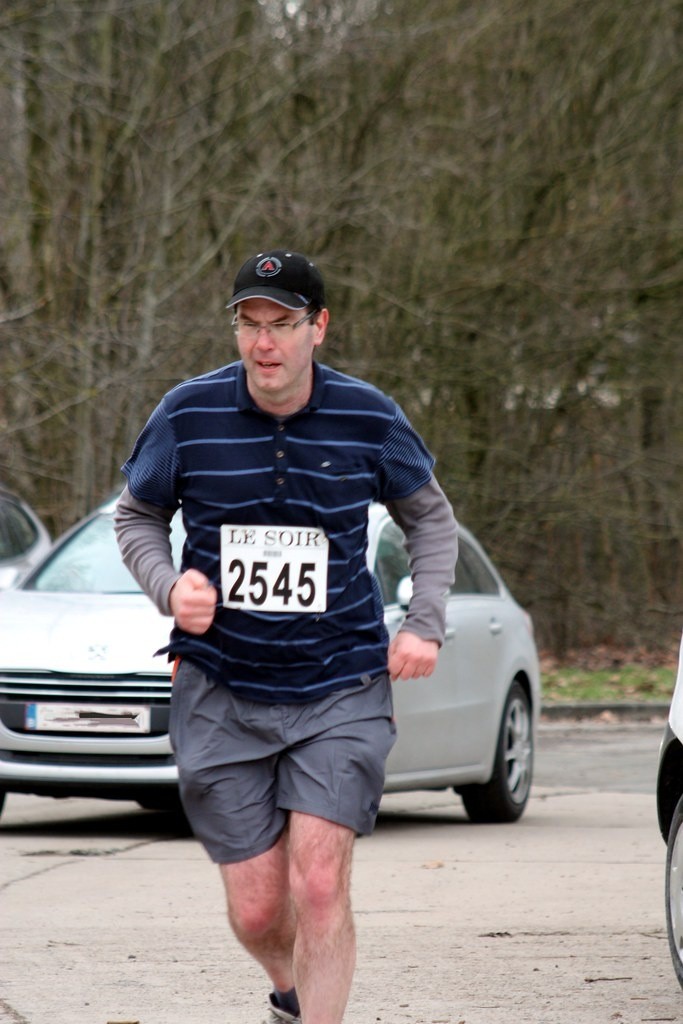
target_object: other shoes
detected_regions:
[268,991,301,1024]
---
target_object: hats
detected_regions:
[225,249,326,311]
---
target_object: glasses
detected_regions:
[231,308,321,336]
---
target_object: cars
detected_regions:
[0,490,540,825]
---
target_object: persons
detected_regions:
[115,250,459,1024]
